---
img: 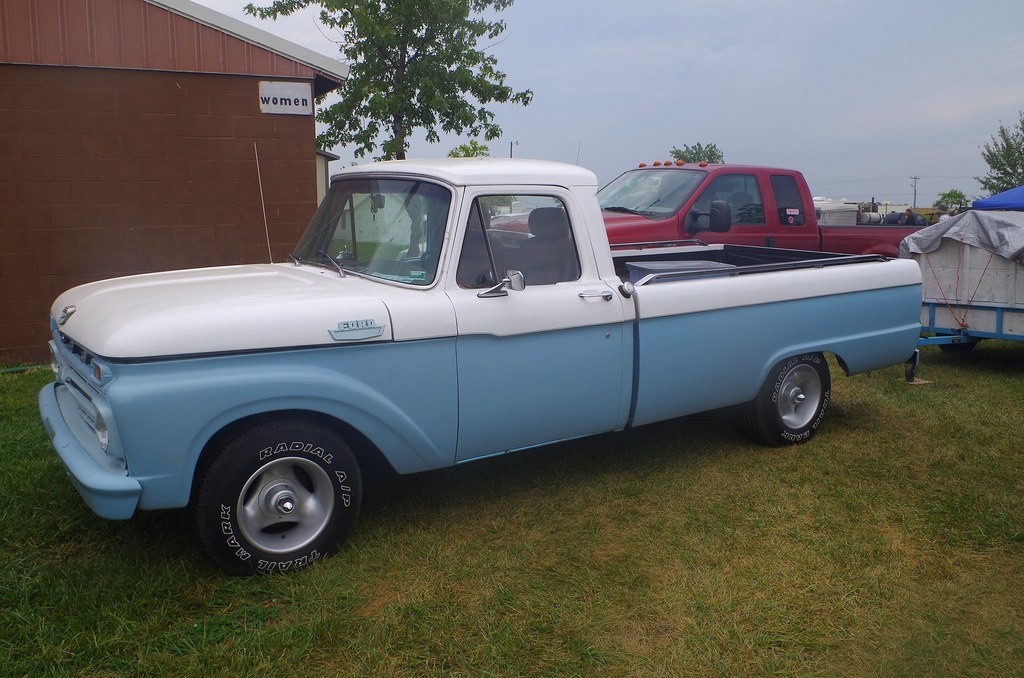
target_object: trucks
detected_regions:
[899,209,1024,363]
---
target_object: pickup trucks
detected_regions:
[37,155,926,580]
[489,159,928,260]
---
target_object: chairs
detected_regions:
[731,192,755,223]
[517,206,577,286]
[697,182,718,226]
[445,202,504,286]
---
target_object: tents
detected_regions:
[972,185,1024,212]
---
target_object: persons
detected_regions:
[936,204,951,222]
[905,208,916,225]
